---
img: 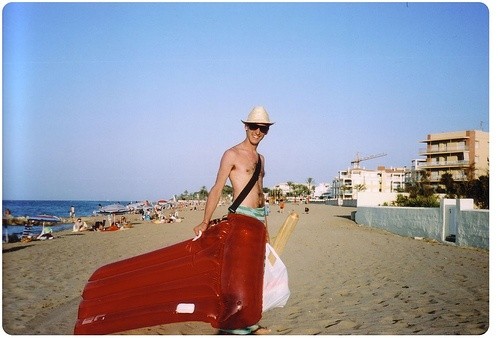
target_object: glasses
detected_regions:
[247,123,269,134]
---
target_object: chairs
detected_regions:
[18,224,33,240]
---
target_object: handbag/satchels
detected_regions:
[262,243,290,312]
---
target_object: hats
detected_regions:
[241,106,274,126]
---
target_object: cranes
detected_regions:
[350,150,388,166]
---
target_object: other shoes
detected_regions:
[218,323,266,335]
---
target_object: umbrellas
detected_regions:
[96,204,127,226]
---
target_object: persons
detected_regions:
[36,190,311,241]
[193,106,274,334]
